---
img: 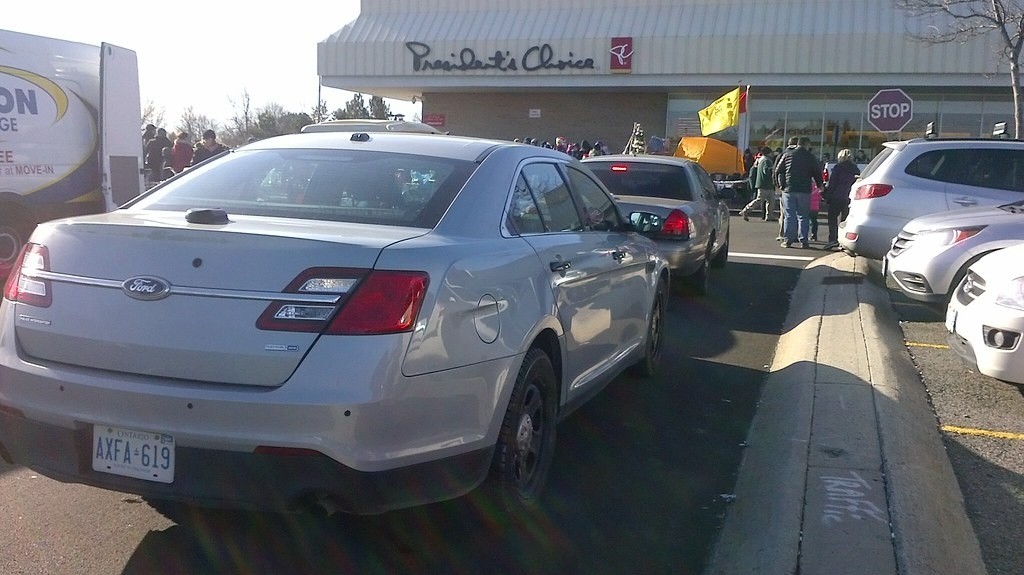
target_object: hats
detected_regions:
[756,153,762,158]
[581,140,590,149]
[146,124,157,131]
[203,130,216,138]
[745,149,750,153]
[573,143,580,148]
[594,143,601,150]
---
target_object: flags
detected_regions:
[698,87,746,137]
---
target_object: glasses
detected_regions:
[203,138,210,139]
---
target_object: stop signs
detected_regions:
[866,89,913,132]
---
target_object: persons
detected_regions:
[142,124,260,181]
[823,148,860,250]
[739,136,829,248]
[514,136,610,160]
[854,150,869,163]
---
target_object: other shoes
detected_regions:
[767,219,778,221]
[809,237,817,241]
[780,242,790,248]
[739,211,749,221]
[800,243,809,248]
[824,241,839,250]
[775,237,780,241]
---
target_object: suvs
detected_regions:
[824,134,1024,388]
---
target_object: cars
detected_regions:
[520,154,730,298]
[0,133,672,544]
